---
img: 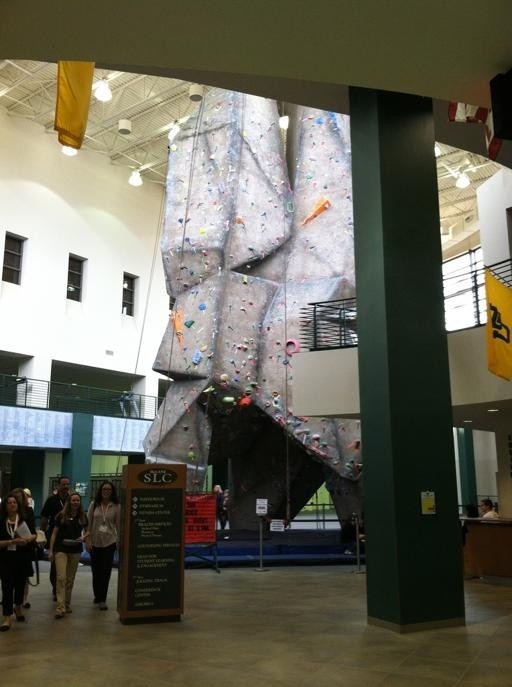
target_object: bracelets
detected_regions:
[9,538,13,545]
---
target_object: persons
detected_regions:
[84,480,122,610]
[0,487,36,608]
[476,498,501,519]
[45,490,91,620]
[38,475,72,603]
[22,486,37,510]
[220,487,232,530]
[0,492,39,631]
[338,514,366,555]
[213,483,225,529]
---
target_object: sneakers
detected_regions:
[94,598,107,610]
[52,588,71,617]
[0,600,30,631]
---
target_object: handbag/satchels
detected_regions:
[30,540,44,561]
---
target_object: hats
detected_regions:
[24,488,31,496]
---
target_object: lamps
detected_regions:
[117,115,133,135]
[188,83,205,102]
[454,168,476,189]
